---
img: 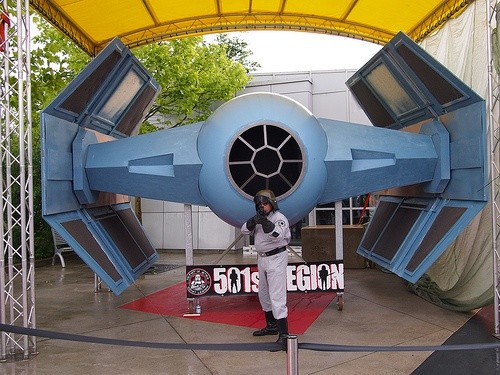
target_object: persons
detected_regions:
[239,189,290,352]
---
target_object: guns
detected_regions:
[254,202,265,215]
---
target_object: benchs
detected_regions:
[51,227,74,268]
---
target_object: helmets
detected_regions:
[254,190,280,210]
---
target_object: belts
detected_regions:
[260,246,286,256]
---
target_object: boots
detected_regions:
[270,317,288,352]
[253,311,278,336]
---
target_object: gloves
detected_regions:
[246,213,266,231]
[260,217,275,234]
